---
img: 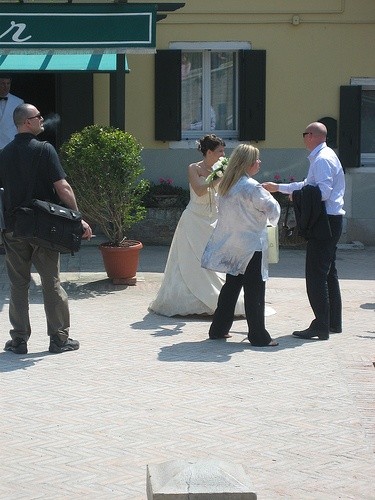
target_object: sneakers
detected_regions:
[5,338,27,354]
[48,338,79,353]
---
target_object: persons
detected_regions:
[201,143,281,346]
[261,122,346,340]
[0,71,24,254]
[148,134,276,317]
[0,103,92,354]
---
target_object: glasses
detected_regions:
[24,114,41,125]
[302,132,312,137]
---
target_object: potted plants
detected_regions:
[58,122,150,279]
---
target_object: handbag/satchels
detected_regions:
[13,199,82,254]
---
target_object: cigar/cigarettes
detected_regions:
[92,235,95,237]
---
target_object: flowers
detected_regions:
[205,156,231,212]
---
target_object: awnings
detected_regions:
[0,54,130,73]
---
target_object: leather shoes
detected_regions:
[294,328,329,339]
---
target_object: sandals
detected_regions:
[264,339,279,346]
[209,332,232,338]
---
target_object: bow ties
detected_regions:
[0,97,8,101]
